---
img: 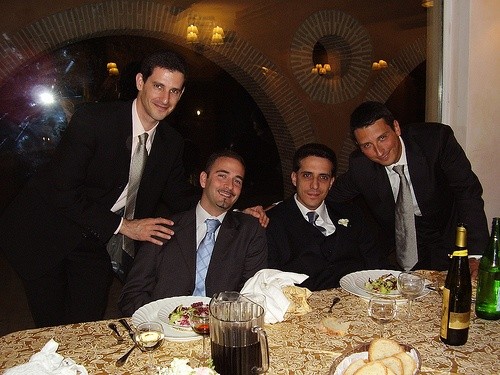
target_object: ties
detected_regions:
[123,133,149,258]
[191,219,221,297]
[393,165,418,272]
[306,212,326,232]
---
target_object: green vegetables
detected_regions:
[366,274,398,294]
[169,304,201,323]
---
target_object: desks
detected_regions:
[0,270,500,375]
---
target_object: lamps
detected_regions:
[107,63,119,75]
[186,24,226,45]
[372,59,388,70]
[312,63,333,79]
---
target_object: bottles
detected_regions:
[440,223,472,346]
[474,217,500,321]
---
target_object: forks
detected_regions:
[108,323,126,342]
[323,297,340,313]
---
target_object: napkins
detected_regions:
[3,338,88,375]
[159,357,220,375]
[240,269,310,324]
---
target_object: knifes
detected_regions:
[120,318,147,353]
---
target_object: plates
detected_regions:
[354,272,401,297]
[158,301,195,327]
[132,296,212,341]
[340,270,433,304]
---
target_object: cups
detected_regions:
[242,293,267,315]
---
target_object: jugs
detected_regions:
[208,290,269,375]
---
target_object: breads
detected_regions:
[342,337,416,375]
[322,317,351,337]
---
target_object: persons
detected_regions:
[13,50,491,329]
[265,143,364,292]
[115,148,268,318]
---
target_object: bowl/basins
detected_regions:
[328,342,422,375]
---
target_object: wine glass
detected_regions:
[133,321,165,370]
[397,272,425,320]
[368,295,397,338]
[190,304,212,358]
